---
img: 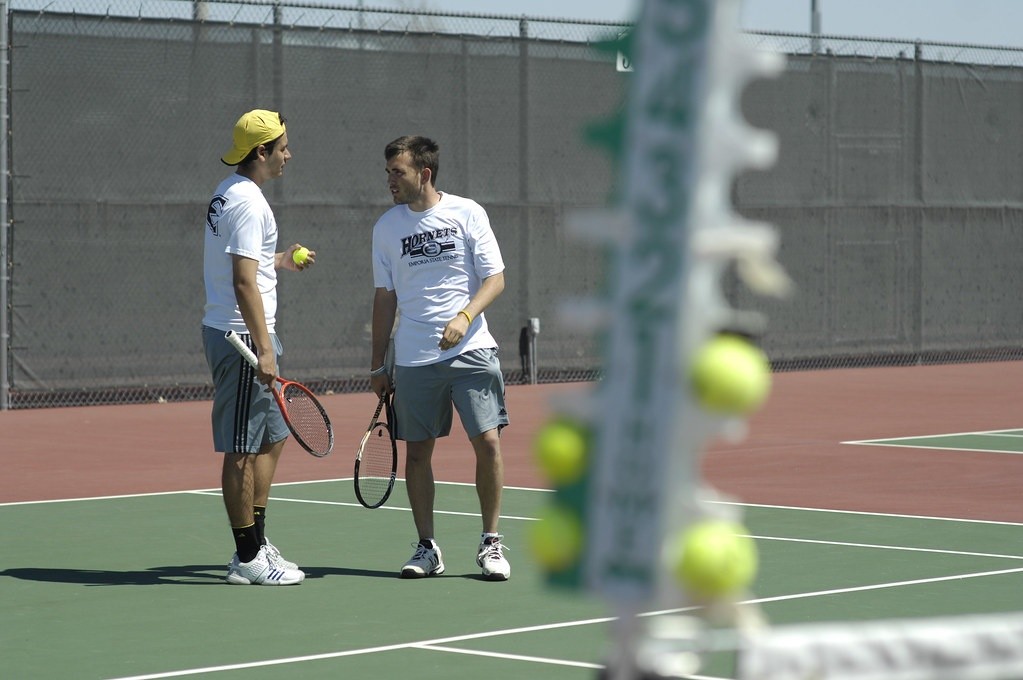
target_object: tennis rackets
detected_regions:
[354,332,398,509]
[224,328,335,457]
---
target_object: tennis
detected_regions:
[693,335,768,419]
[292,246,310,266]
[531,422,590,487]
[676,519,757,602]
[527,505,588,569]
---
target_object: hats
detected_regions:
[221,109,286,166]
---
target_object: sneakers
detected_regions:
[401,542,446,576]
[227,535,298,569]
[226,545,305,584]
[475,535,511,579]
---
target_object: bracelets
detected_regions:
[369,366,385,376]
[457,310,472,327]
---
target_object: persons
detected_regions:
[369,135,511,580]
[202,108,305,585]
